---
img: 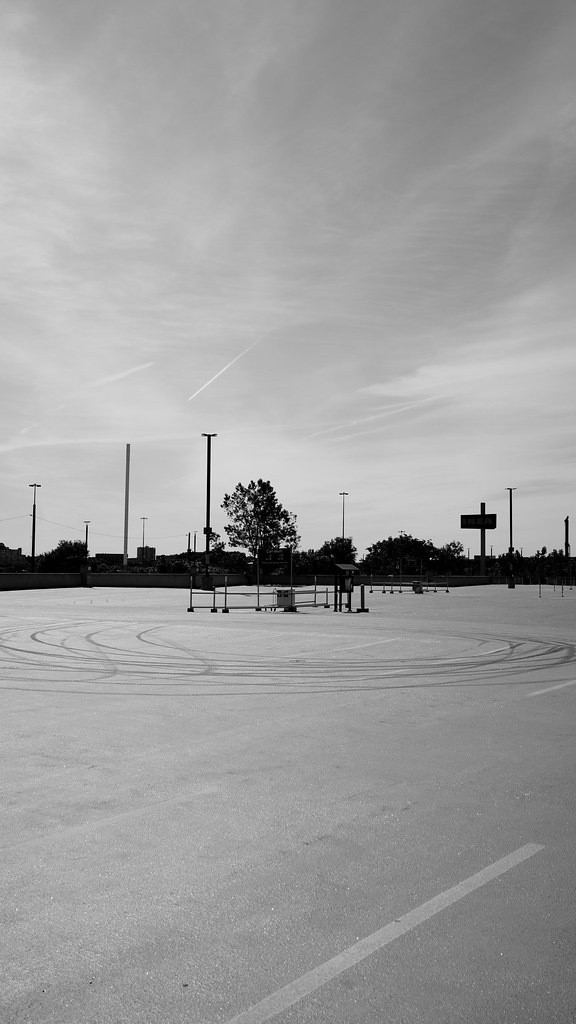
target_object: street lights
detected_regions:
[29,483,41,573]
[83,521,91,562]
[505,487,517,554]
[339,492,348,539]
[202,433,217,572]
[140,516,147,566]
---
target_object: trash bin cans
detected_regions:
[277,589,295,607]
[412,580,422,591]
